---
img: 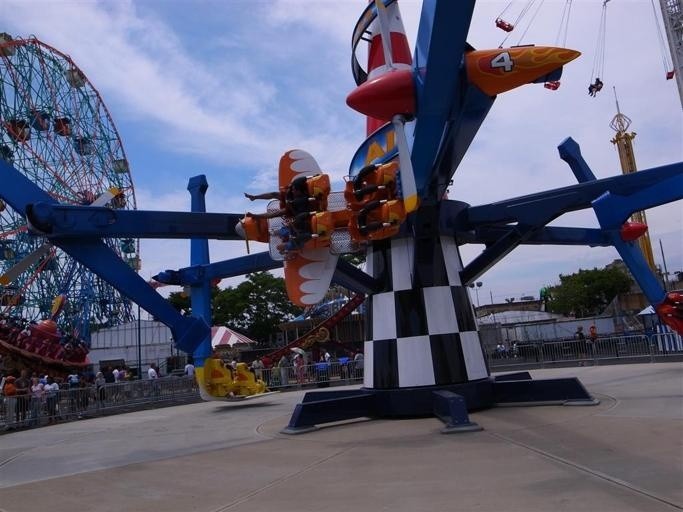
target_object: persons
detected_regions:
[591,81,602,97]
[512,340,519,358]
[0,365,131,432]
[496,341,505,360]
[270,218,313,256]
[270,348,365,389]
[154,367,161,378]
[251,355,264,379]
[585,324,596,355]
[184,360,195,392]
[231,357,237,370]
[503,338,510,360]
[145,362,160,397]
[242,183,303,220]
[587,77,598,96]
[573,325,586,366]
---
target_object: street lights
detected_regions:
[469,281,483,321]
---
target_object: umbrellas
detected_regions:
[288,346,305,355]
